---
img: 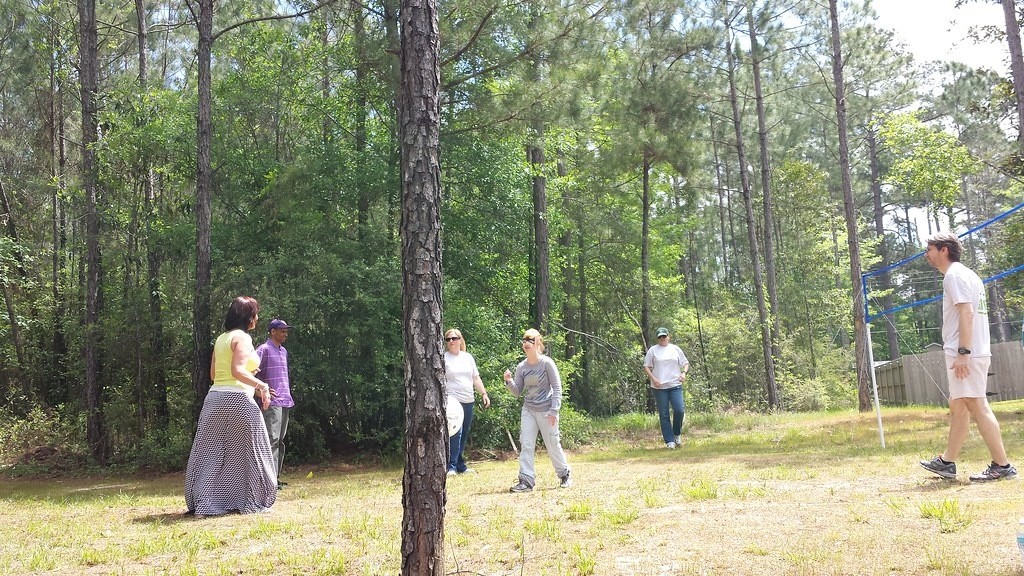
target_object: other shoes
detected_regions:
[666,442,675,450]
[465,468,475,473]
[447,471,456,475]
[674,436,681,445]
[277,480,288,490]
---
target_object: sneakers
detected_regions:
[920,456,956,479]
[510,478,533,493]
[560,470,572,489]
[969,461,1017,483]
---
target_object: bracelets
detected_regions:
[482,393,488,397]
[255,378,260,389]
[260,381,270,393]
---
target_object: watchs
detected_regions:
[681,371,688,375]
[958,347,971,355]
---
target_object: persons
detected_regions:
[503,328,572,493]
[643,327,690,451]
[918,232,1018,483]
[444,329,490,479]
[184,295,279,520]
[253,318,295,490]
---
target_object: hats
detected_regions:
[656,327,668,338]
[446,396,464,437]
[267,319,293,333]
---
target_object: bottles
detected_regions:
[1017,519,1024,567]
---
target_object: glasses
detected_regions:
[925,248,932,252]
[445,337,460,342]
[522,337,535,344]
[658,335,666,339]
